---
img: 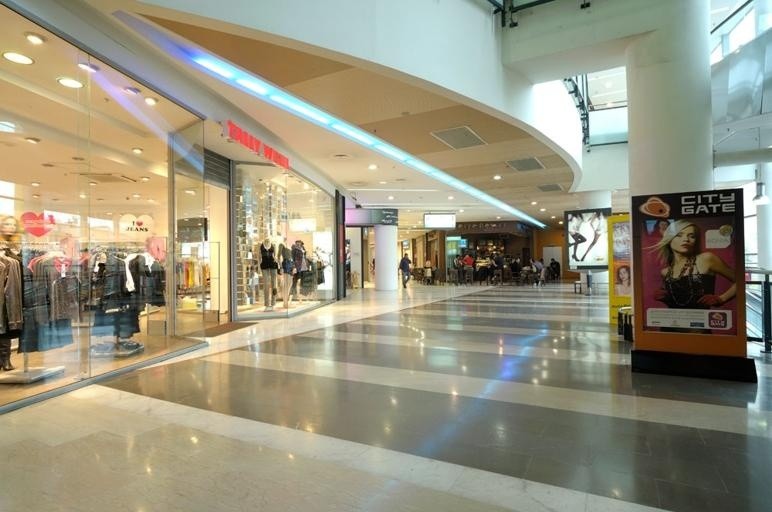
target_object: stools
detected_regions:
[574,280,583,295]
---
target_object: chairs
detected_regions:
[411,258,564,290]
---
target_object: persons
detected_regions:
[614,266,631,297]
[453,249,560,288]
[650,220,670,239]
[398,254,411,287]
[580,212,608,261]
[568,214,586,261]
[255,235,309,311]
[642,220,737,309]
[0,216,22,248]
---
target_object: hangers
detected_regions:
[0,238,148,264]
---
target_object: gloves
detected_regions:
[697,294,726,310]
[654,288,668,302]
